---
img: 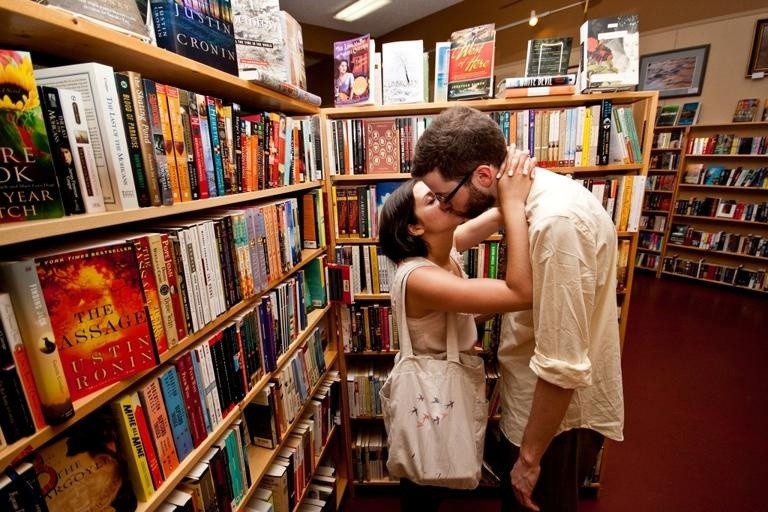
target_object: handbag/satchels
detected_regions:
[379,261,488,488]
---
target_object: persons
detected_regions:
[334,59,356,100]
[379,144,538,512]
[411,105,625,511]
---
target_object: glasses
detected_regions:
[433,166,477,204]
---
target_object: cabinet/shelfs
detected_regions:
[634,125,689,279]
[0,0,354,512]
[321,90,660,502]
[658,121,768,294]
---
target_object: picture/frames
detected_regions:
[744,18,768,79]
[566,64,579,86]
[634,43,711,101]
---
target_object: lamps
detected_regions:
[528,10,539,27]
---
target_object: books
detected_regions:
[326,103,645,174]
[0,189,343,447]
[0,47,333,224]
[343,303,399,349]
[334,243,399,297]
[657,103,680,125]
[649,131,686,151]
[731,99,758,122]
[636,213,671,233]
[152,1,238,77]
[230,1,323,107]
[682,162,768,189]
[332,183,379,239]
[670,225,768,258]
[642,194,671,210]
[573,175,646,232]
[618,239,630,291]
[434,23,497,104]
[674,196,767,223]
[663,253,767,291]
[331,34,430,104]
[645,174,680,191]
[461,242,500,279]
[41,0,153,42]
[353,433,391,481]
[678,102,703,125]
[345,359,391,419]
[496,14,640,99]
[632,235,664,251]
[687,133,767,155]
[473,312,503,349]
[648,155,682,172]
[483,357,503,417]
[0,419,341,512]
[635,253,661,268]
